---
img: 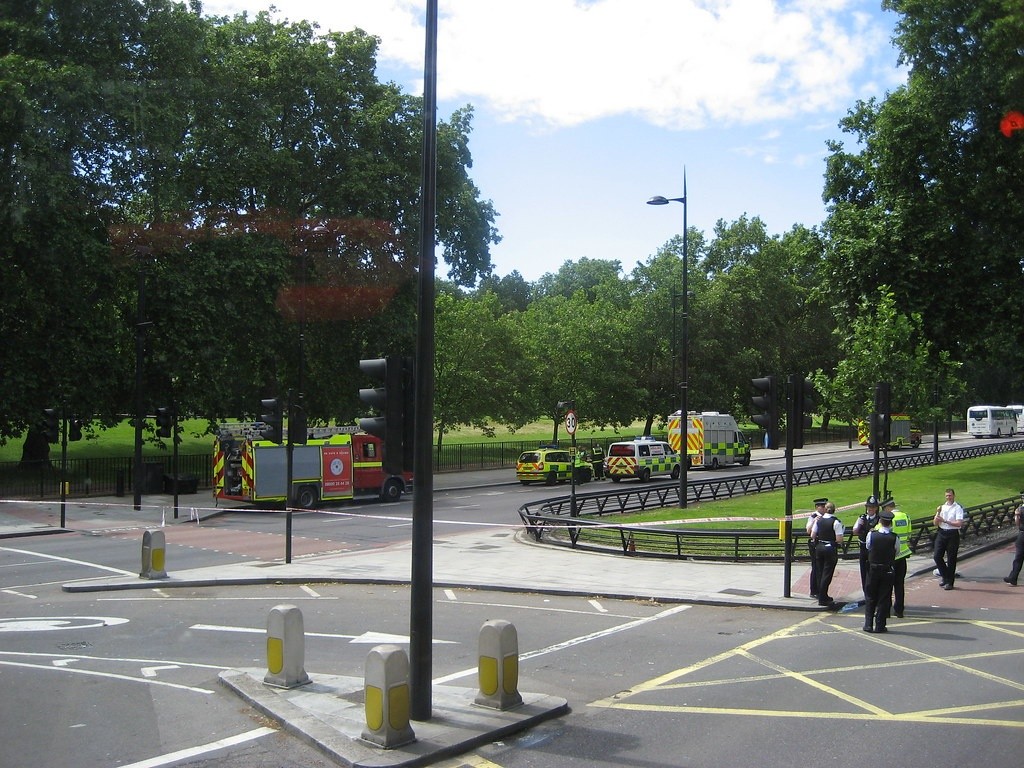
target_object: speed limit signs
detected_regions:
[564,410,578,435]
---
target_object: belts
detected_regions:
[940,528,958,531]
[818,540,834,543]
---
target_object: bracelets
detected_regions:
[944,519,947,523]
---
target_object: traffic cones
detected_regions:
[627,533,636,552]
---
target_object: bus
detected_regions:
[1006,406,1024,434]
[966,406,1019,438]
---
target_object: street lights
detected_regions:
[648,195,687,509]
[672,291,696,413]
[295,224,329,408]
[134,322,153,510]
[557,400,576,535]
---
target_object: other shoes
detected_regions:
[894,610,903,618]
[863,626,873,632]
[810,595,819,600]
[876,627,887,632]
[818,597,833,605]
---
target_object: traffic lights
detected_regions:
[751,379,778,450]
[67,419,83,441]
[999,107,1024,142]
[359,355,412,480]
[154,406,171,439]
[260,398,284,445]
[41,407,58,442]
[794,379,815,450]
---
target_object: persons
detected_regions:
[805,498,829,598]
[862,510,901,633]
[933,488,962,591]
[811,502,844,606]
[853,495,882,601]
[1004,490,1024,585]
[872,496,912,617]
[572,443,605,483]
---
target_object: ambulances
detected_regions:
[668,411,752,469]
[603,436,680,482]
[516,444,594,486]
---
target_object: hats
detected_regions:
[879,497,895,507]
[813,498,828,504]
[865,496,878,506]
[879,510,895,521]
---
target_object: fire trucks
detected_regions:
[211,435,413,506]
[856,415,923,451]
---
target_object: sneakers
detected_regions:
[1004,577,1017,587]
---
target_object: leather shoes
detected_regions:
[939,581,953,590]
